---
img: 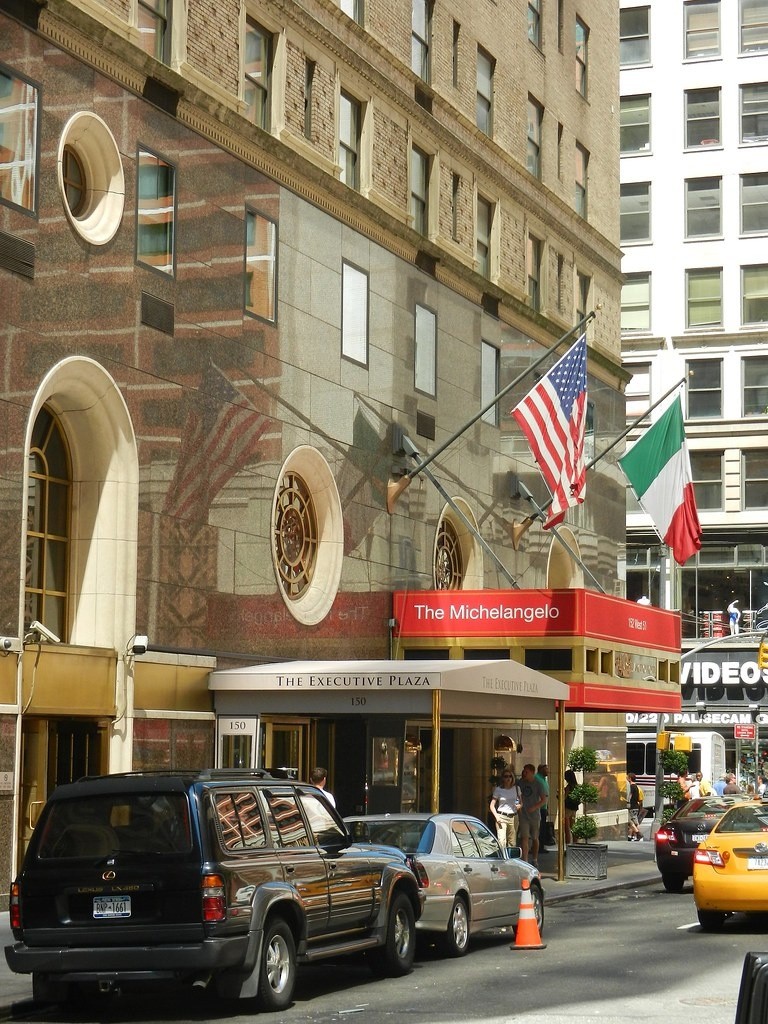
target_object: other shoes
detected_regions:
[628,837,631,841]
[631,837,635,839]
[634,834,643,841]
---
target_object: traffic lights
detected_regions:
[758,641,768,670]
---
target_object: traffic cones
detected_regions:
[503,879,546,950]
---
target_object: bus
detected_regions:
[626,732,725,813]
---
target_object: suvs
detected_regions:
[3,765,427,1015]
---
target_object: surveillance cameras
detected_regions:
[132,636,148,654]
[30,621,60,644]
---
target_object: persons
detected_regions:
[557,769,582,855]
[728,600,740,636]
[637,596,650,606]
[689,772,766,801]
[626,772,644,841]
[533,763,550,853]
[518,764,545,867]
[309,768,335,813]
[490,770,523,848]
[677,770,695,809]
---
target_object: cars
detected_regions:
[654,796,759,892]
[339,813,545,957]
[690,799,767,930]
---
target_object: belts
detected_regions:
[497,811,518,817]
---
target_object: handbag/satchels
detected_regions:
[543,821,557,846]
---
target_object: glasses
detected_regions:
[503,775,512,778]
[733,777,736,780]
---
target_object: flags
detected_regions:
[158,357,273,540]
[616,392,703,568]
[508,332,588,528]
[330,408,409,562]
